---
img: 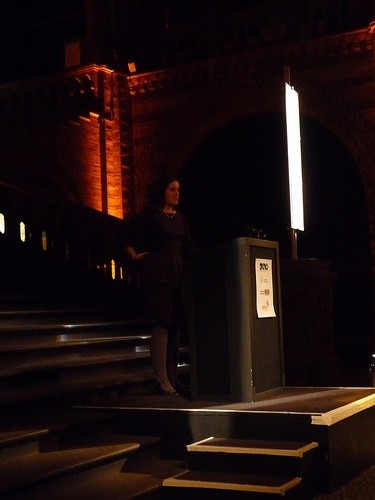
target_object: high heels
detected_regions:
[155,378,179,397]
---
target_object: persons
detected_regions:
[122,171,198,400]
